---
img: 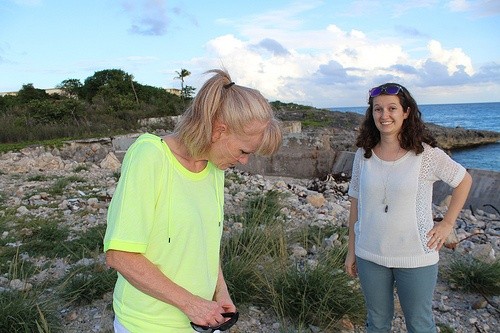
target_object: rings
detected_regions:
[437,237,440,242]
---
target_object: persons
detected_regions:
[103,68,283,333]
[344,82,473,332]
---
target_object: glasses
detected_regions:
[369,85,403,97]
[190,311,239,333]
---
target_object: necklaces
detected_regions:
[377,143,402,213]
